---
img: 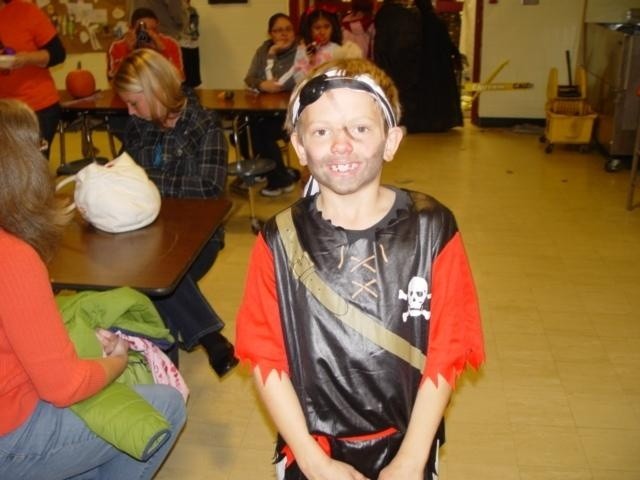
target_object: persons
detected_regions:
[227,12,302,197]
[76,48,242,379]
[236,60,486,480]
[107,7,186,89]
[0,98,191,480]
[164,1,202,93]
[1,0,67,161]
[292,8,363,83]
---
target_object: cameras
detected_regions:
[135,21,151,44]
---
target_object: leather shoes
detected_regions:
[210,343,240,376]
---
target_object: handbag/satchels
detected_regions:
[75,152,162,235]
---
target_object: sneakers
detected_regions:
[261,174,295,196]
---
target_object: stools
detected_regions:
[226,159,282,234]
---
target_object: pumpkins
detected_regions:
[65,61,96,98]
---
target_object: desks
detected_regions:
[58,88,292,167]
[27,173,232,298]
